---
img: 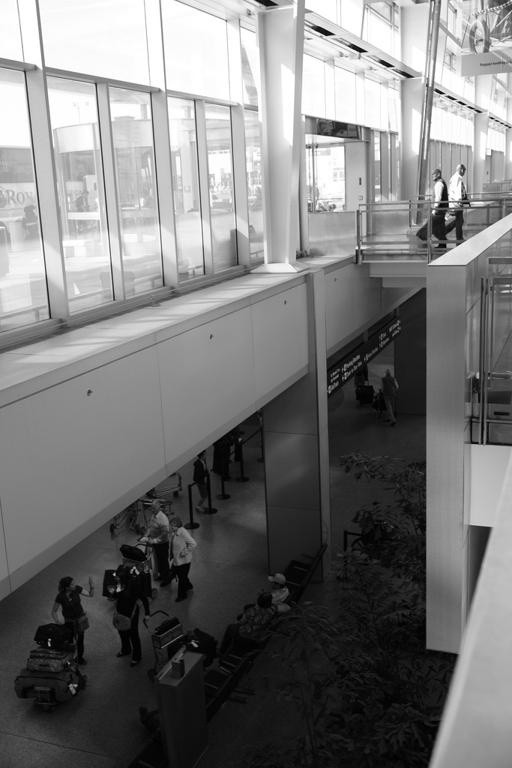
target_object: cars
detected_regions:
[211,185,258,213]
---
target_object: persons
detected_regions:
[355,363,368,400]
[167,517,198,603]
[116,574,150,667]
[51,577,95,665]
[236,573,291,621]
[139,500,171,587]
[430,168,449,248]
[445,163,468,246]
[381,368,400,426]
[218,591,273,653]
[193,450,209,513]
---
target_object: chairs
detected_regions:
[138,541,333,734]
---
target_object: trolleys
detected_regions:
[149,472,185,499]
[142,609,188,684]
[372,393,393,416]
[137,496,182,540]
[30,620,90,712]
[112,540,159,602]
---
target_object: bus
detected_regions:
[1,144,101,251]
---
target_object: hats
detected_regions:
[267,573,286,585]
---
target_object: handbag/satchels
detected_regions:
[119,543,146,561]
[112,607,131,631]
[75,615,89,632]
[32,621,76,644]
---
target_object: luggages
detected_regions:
[355,384,374,400]
[151,616,187,679]
[27,642,75,671]
[12,666,80,702]
[102,556,152,599]
